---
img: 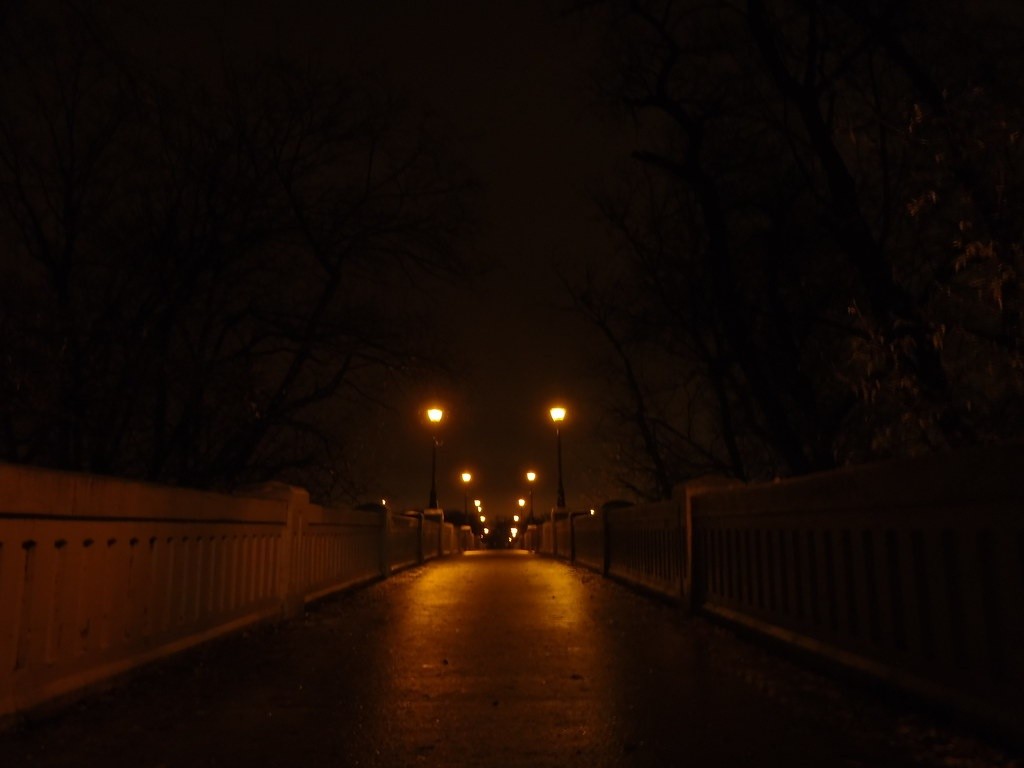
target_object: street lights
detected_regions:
[427,407,442,508]
[461,473,471,525]
[509,498,525,543]
[527,472,536,523]
[474,497,489,545]
[550,406,569,508]
